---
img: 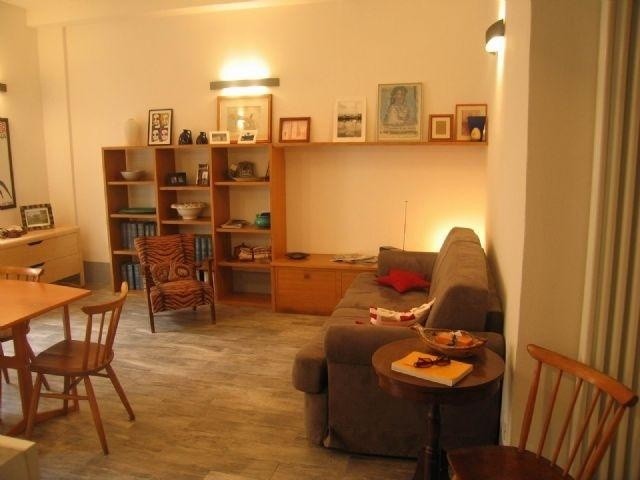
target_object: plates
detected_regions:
[119,208,157,214]
[1,229,27,238]
[231,178,267,181]
[285,253,311,260]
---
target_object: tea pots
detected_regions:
[196,132,209,144]
[178,129,192,145]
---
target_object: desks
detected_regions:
[0,280,92,437]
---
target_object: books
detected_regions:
[118,221,214,291]
[195,163,210,186]
[117,207,156,216]
[390,350,474,387]
[220,219,247,229]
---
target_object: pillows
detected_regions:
[369,297,436,328]
[375,268,430,293]
[147,260,194,285]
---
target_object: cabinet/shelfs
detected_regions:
[271,253,378,317]
[0,225,85,287]
[102,141,287,307]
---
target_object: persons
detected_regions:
[150,113,169,143]
[383,85,416,129]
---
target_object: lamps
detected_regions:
[486,19,505,54]
[210,77,280,90]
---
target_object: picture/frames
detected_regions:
[20,204,55,232]
[0,118,16,210]
[333,96,366,142]
[210,94,272,144]
[428,114,454,142]
[279,117,311,143]
[147,109,173,145]
[375,82,423,143]
[455,104,487,142]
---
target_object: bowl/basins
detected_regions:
[169,201,208,220]
[120,171,145,181]
[409,322,488,358]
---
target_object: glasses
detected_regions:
[414,354,450,368]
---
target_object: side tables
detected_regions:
[372,338,506,480]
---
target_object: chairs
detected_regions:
[134,233,216,333]
[0,265,50,391]
[25,281,135,455]
[446,345,640,480]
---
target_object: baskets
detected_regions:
[419,327,488,357]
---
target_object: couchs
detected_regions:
[292,227,505,459]
